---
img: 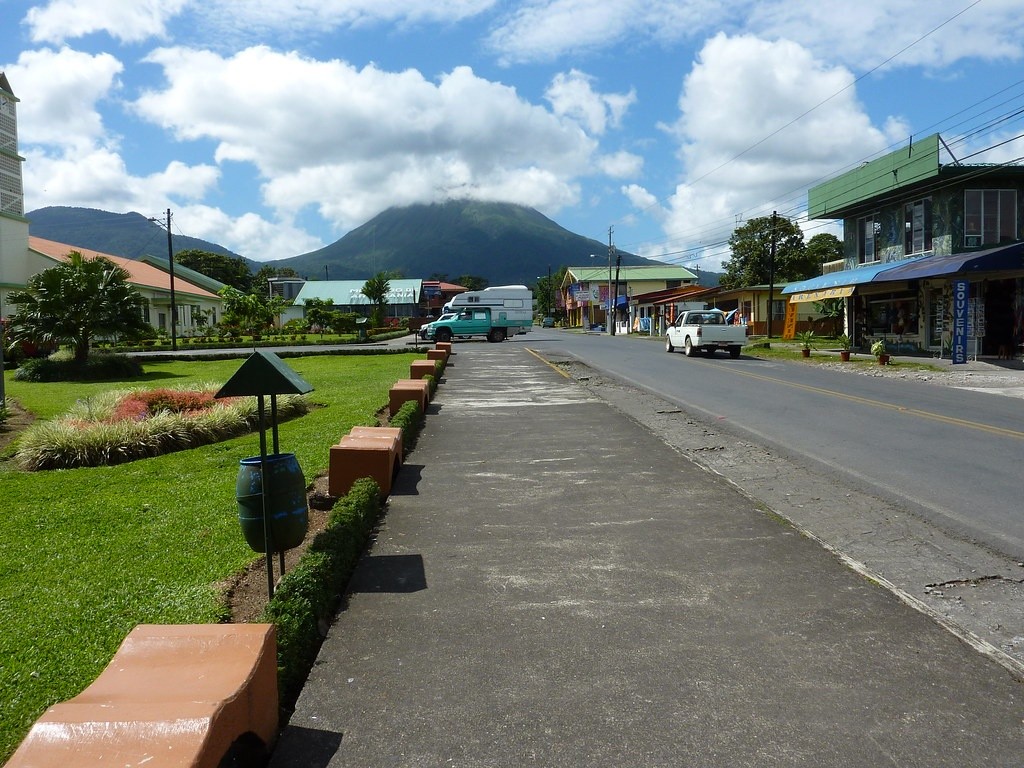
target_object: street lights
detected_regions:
[536,264,551,317]
[147,216,178,351]
[590,254,614,335]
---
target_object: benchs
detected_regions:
[410,360,436,379]
[427,349,446,361]
[388,378,430,415]
[0,624,280,768]
[329,425,403,496]
[436,342,451,354]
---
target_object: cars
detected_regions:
[541,317,556,328]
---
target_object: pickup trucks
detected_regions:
[419,284,534,343]
[665,309,747,359]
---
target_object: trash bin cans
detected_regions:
[356,318,368,337]
[214,348,315,553]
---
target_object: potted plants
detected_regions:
[796,330,817,357]
[836,333,852,362]
[871,340,890,365]
